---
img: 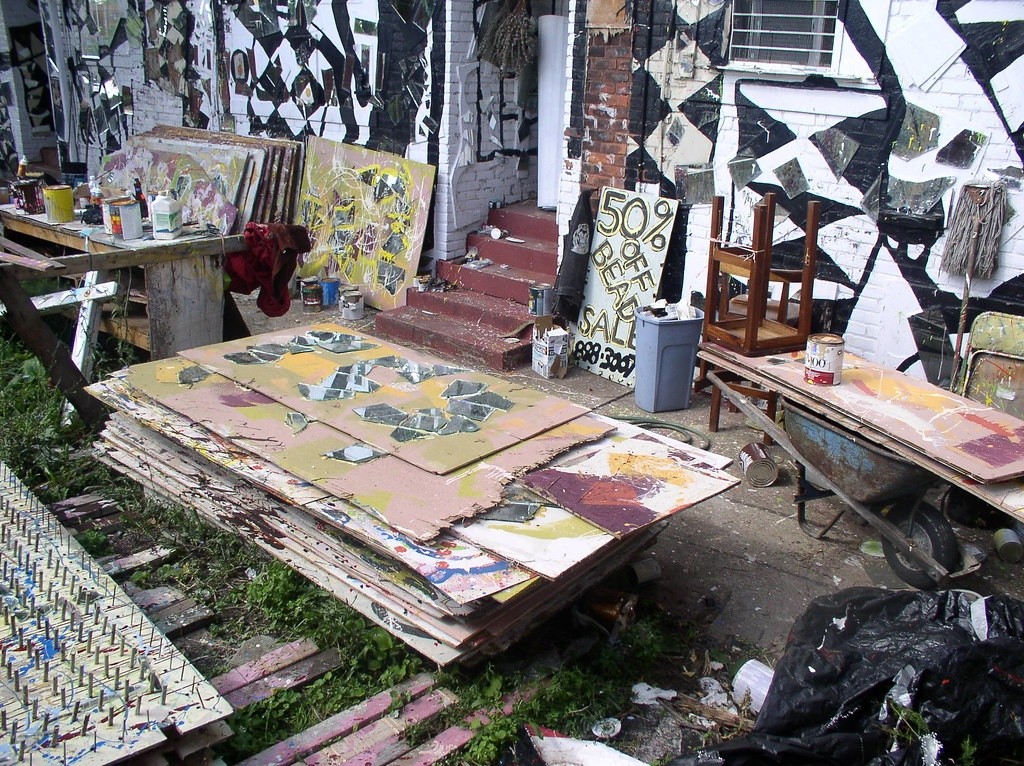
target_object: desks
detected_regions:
[0,203,246,361]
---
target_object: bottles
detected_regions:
[126,178,183,240]
[94,189,104,198]
[89,176,98,189]
[18,156,28,178]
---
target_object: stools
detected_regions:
[702,192,821,359]
[708,294,800,444]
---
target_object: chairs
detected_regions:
[954,312,1024,422]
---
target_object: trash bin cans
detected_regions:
[632,303,705,415]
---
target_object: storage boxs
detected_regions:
[7,178,48,215]
[531,313,569,381]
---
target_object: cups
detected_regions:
[413,275,432,293]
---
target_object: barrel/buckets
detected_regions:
[42,185,74,224]
[11,179,45,214]
[738,442,779,488]
[321,278,341,305]
[101,197,124,234]
[338,285,359,313]
[804,334,845,387]
[109,198,144,240]
[341,290,365,320]
[301,276,321,305]
[528,283,552,317]
